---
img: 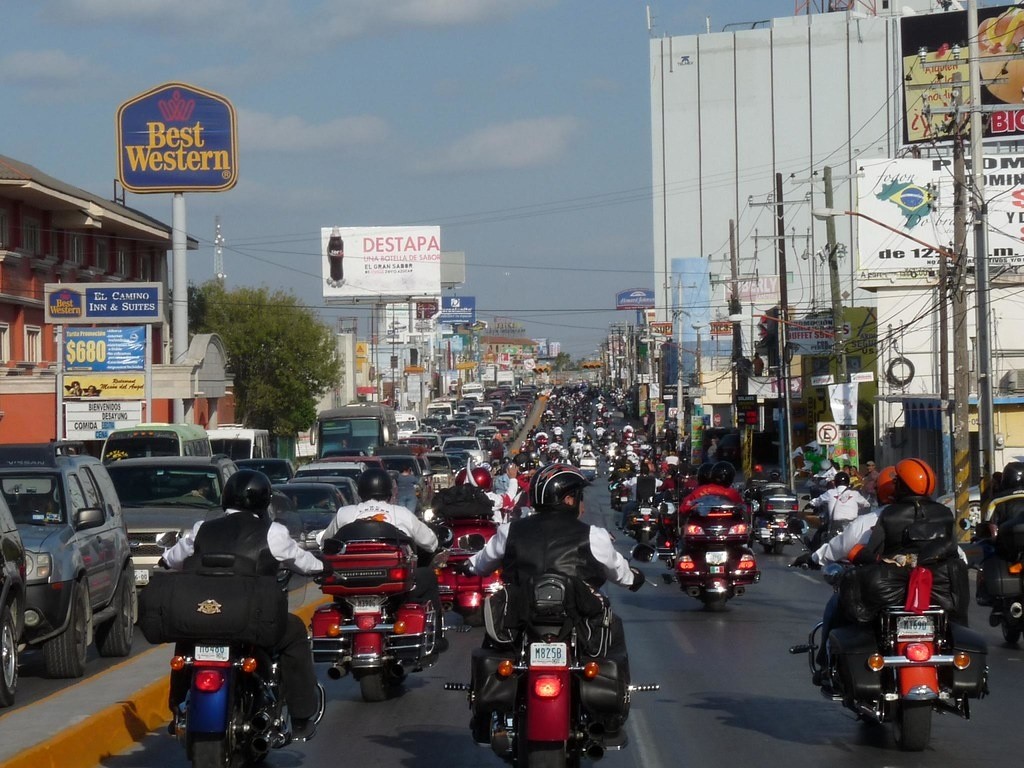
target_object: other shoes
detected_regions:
[989,599,1006,627]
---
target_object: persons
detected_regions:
[156,469,334,731]
[189,476,216,499]
[450,462,646,739]
[448,390,879,550]
[342,437,350,447]
[989,471,1002,495]
[791,465,898,686]
[819,456,972,694]
[318,470,450,654]
[311,496,336,509]
[401,467,414,475]
[970,462,1024,617]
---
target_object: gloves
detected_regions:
[794,554,814,567]
[629,567,646,592]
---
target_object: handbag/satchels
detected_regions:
[140,568,288,646]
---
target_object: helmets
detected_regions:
[469,469,492,491]
[696,461,736,488]
[626,444,633,451]
[222,471,272,512]
[835,472,850,486]
[530,465,591,506]
[479,420,615,475]
[1002,462,1024,490]
[358,469,393,497]
[876,458,935,504]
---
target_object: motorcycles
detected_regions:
[307,520,460,705]
[429,387,869,629]
[153,529,348,768]
[789,557,994,754]
[957,515,1024,645]
[443,541,661,768]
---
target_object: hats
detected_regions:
[753,465,763,473]
[770,469,781,478]
[866,461,875,465]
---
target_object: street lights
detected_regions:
[640,337,703,388]
[727,311,850,384]
[812,207,980,546]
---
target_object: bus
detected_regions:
[99,425,213,464]
[309,406,400,460]
[203,425,271,463]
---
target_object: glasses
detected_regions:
[571,492,585,502]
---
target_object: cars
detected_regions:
[933,483,982,527]
[0,382,539,709]
